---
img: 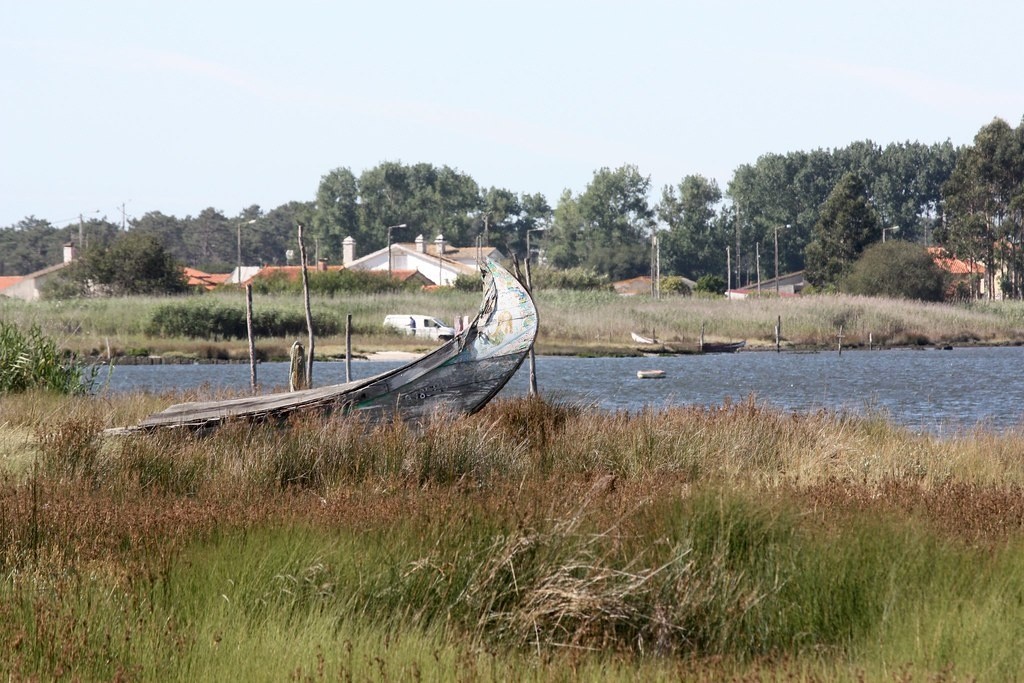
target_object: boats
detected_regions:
[630,330,746,356]
[89,253,538,464]
[637,369,666,380]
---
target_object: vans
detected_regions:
[382,314,455,344]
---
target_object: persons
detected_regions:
[405,317,416,336]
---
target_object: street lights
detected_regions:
[236,220,258,287]
[774,224,792,294]
[388,224,408,279]
[883,226,899,245]
[526,227,545,258]
